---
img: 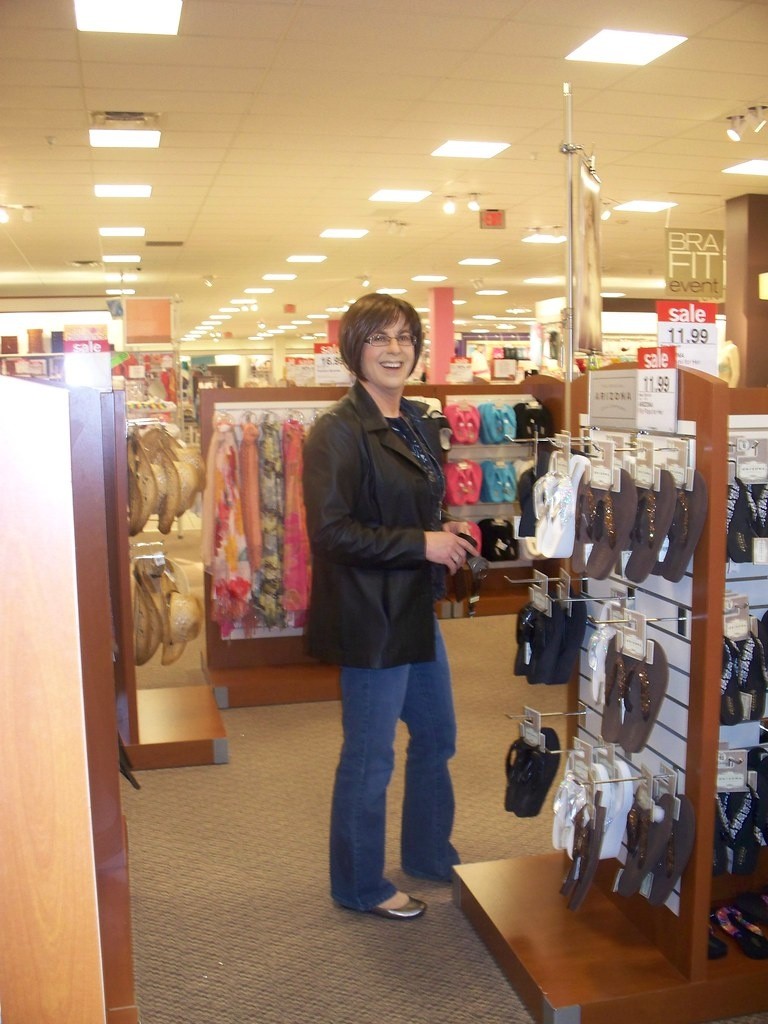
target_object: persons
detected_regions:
[301,293,479,918]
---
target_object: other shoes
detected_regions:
[341,895,427,920]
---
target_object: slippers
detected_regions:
[503,593,768,960]
[442,457,534,561]
[525,454,768,584]
[423,400,554,451]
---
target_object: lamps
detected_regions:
[726,106,768,142]
[442,193,480,215]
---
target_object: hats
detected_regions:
[124,423,206,536]
[131,553,200,666]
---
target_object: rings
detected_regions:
[453,556,460,561]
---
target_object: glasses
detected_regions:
[363,333,418,346]
